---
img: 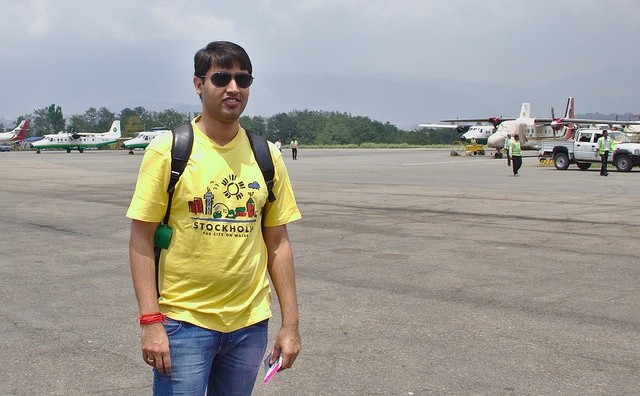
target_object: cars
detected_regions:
[0,143,12,152]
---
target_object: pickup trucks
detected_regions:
[539,128,640,172]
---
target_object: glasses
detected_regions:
[200,71,255,88]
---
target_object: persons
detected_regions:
[504,133,514,166]
[594,130,613,177]
[289,138,298,161]
[509,132,523,177]
[274,138,283,153]
[125,39,303,396]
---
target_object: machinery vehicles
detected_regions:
[451,141,485,157]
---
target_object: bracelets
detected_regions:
[135,312,168,327]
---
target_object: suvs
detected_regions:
[14,137,43,151]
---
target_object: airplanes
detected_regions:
[30,120,132,153]
[461,126,498,144]
[0,119,28,142]
[121,127,171,155]
[441,102,640,158]
